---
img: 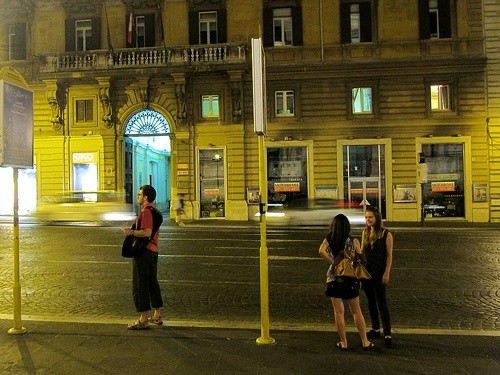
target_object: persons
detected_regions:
[122,185,163,330]
[319,214,376,351]
[360,206,393,342]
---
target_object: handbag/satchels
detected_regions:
[121,205,164,259]
[332,237,373,283]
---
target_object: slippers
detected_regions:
[362,341,376,350]
[336,341,347,350]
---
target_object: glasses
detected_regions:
[136,191,143,197]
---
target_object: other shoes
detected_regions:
[366,329,383,339]
[383,334,392,344]
[151,313,163,327]
[126,319,150,331]
